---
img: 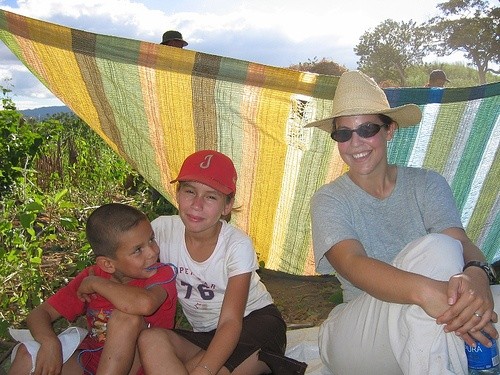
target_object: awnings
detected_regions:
[0,9,500,277]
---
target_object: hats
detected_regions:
[160,31,188,46]
[303,70,421,130]
[431,70,450,82]
[169,150,238,195]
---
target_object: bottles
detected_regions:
[464,330,500,375]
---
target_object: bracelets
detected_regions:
[195,364,214,375]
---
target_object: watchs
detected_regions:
[462,261,496,285]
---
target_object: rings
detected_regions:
[473,312,482,319]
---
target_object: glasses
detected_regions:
[331,123,387,142]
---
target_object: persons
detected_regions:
[159,31,188,49]
[301,70,500,375]
[8,149,287,375]
[425,69,451,87]
